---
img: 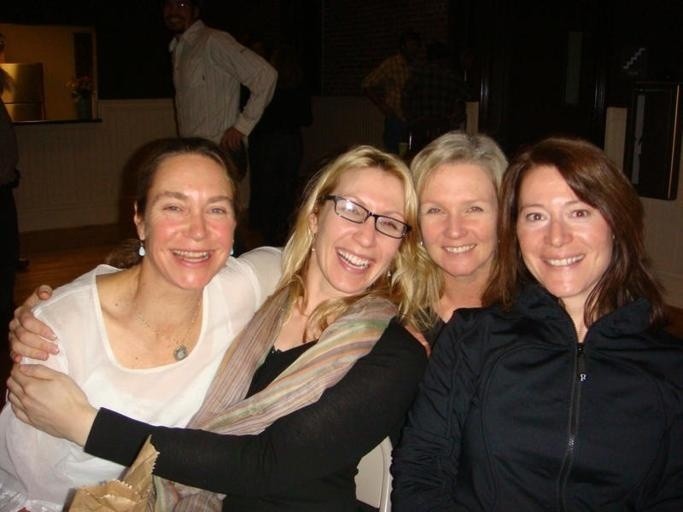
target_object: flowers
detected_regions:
[63,75,95,97]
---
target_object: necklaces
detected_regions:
[295,301,311,318]
[127,293,202,364]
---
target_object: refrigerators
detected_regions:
[0,62,45,121]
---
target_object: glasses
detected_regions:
[321,195,413,240]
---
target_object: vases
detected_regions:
[74,95,90,119]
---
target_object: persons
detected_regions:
[388,127,510,365]
[398,39,480,160]
[388,134,683,512]
[160,0,279,186]
[239,32,314,248]
[0,34,23,372]
[357,32,424,159]
